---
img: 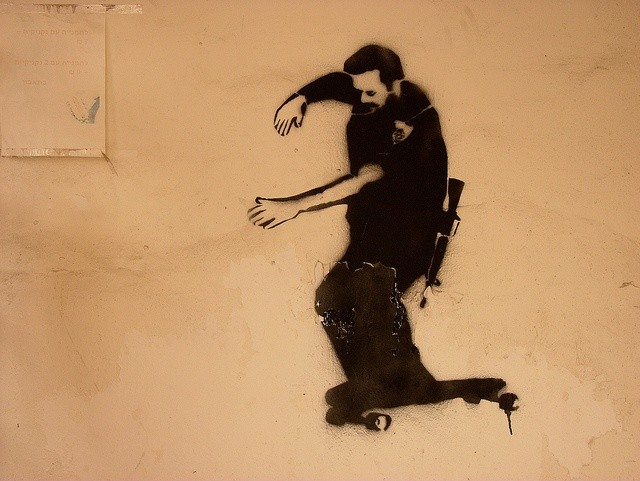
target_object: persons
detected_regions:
[245,46,449,408]
